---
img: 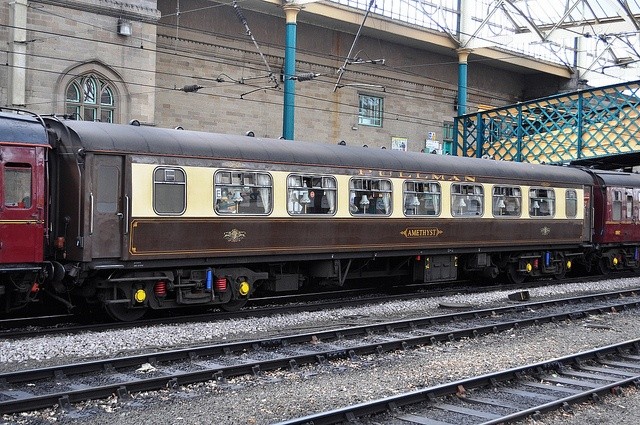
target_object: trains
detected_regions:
[0,106,640,323]
